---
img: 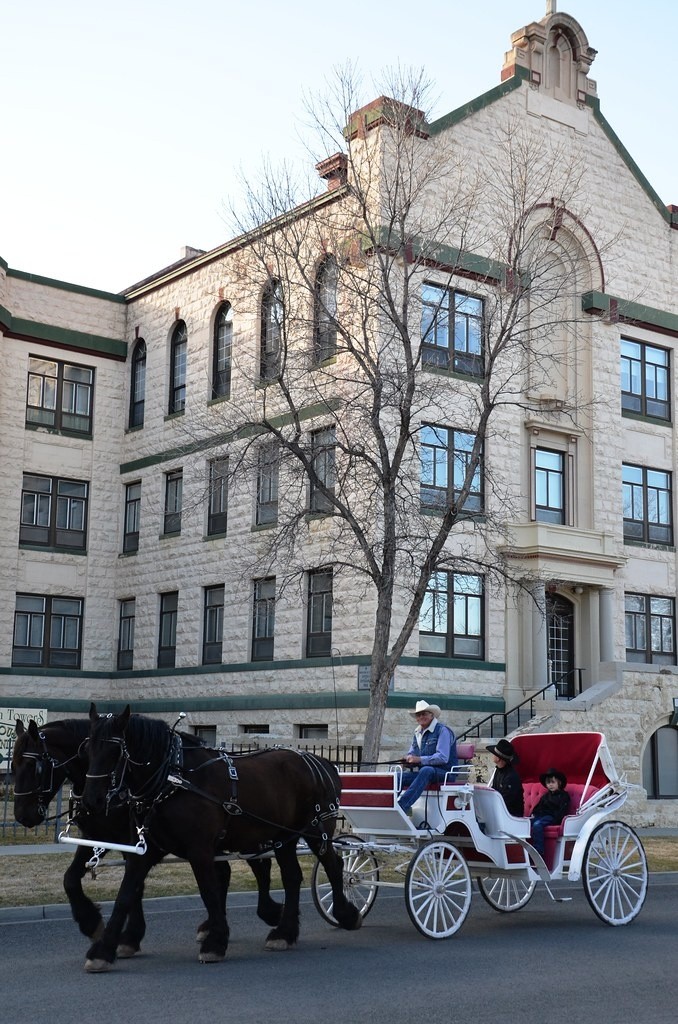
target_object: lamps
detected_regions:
[571,587,584,595]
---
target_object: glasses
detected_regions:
[416,712,427,717]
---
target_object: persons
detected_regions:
[485,739,525,818]
[398,700,460,817]
[530,768,573,869]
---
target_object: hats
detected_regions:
[408,700,441,719]
[539,768,567,790]
[486,739,520,765]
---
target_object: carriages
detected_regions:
[9,703,654,979]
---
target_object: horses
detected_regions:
[13,718,285,959]
[81,702,363,972]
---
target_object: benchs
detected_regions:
[394,744,473,833]
[473,782,600,871]
